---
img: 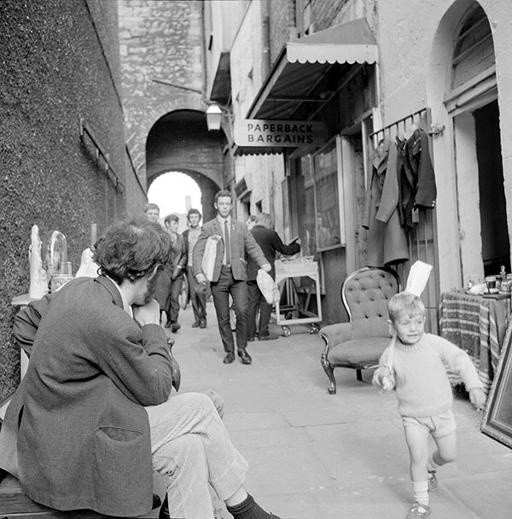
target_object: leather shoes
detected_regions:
[258,333,278,342]
[222,350,235,364]
[192,320,198,327]
[164,322,171,329]
[247,336,255,342]
[172,325,181,333]
[199,316,206,327]
[238,349,252,364]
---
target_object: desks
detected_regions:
[10,292,43,384]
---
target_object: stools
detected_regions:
[1,471,164,518]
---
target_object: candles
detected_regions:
[29,224,48,299]
[90,222,97,249]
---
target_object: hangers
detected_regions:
[376,127,386,149]
[403,113,418,137]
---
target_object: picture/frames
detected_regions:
[480,313,511,448]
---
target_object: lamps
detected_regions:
[206,103,222,131]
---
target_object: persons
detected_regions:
[0,212,281,519]
[135,204,173,325]
[317,213,332,249]
[241,213,301,340]
[370,292,486,515]
[191,190,272,364]
[161,216,187,332]
[181,209,209,328]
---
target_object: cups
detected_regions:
[468,272,485,289]
[60,261,72,276]
[485,275,497,293]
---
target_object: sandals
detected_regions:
[429,468,439,493]
[406,500,431,519]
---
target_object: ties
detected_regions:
[224,220,231,266]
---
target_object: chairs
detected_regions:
[318,266,402,394]
